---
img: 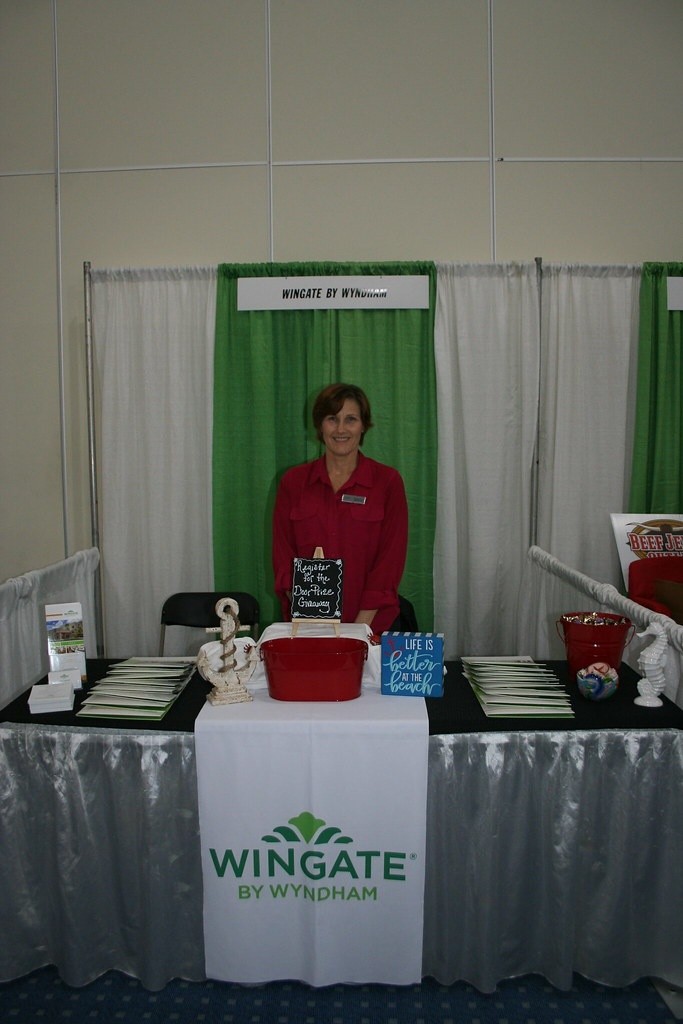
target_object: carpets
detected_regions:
[0,964,678,1024]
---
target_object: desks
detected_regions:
[0,657,682,993]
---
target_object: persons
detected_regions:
[271,382,408,636]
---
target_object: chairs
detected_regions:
[160,592,261,658]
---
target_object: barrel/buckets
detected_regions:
[556,612,635,681]
[260,638,368,702]
